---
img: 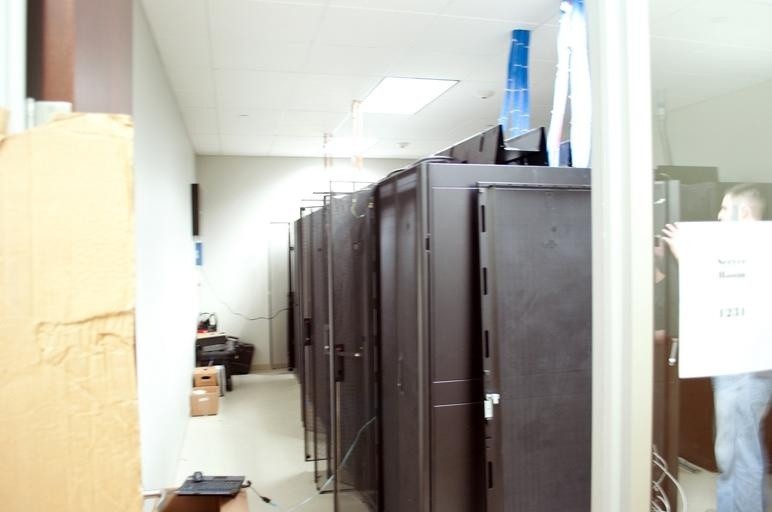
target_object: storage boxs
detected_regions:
[156,487,249,512]
[194,367,217,386]
[191,386,219,416]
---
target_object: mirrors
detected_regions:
[650,1,772,512]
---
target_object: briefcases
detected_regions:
[226,335,254,375]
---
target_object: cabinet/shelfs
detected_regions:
[295,168,592,512]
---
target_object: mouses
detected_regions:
[194,472,202,482]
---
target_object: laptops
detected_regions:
[175,475,244,496]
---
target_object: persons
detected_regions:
[655,183,770,512]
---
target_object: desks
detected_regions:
[201,351,235,391]
